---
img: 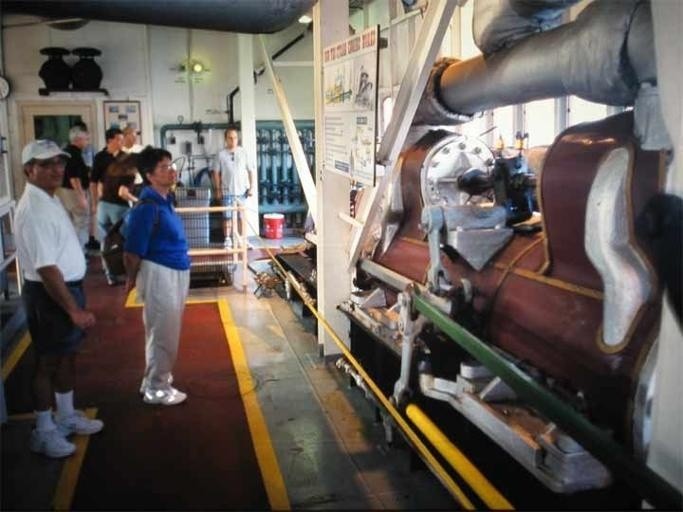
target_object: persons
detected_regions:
[114,121,147,200]
[76,120,101,250]
[214,128,254,250]
[90,128,130,287]
[60,127,91,265]
[15,139,104,459]
[124,145,191,407]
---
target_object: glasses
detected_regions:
[231,152,234,161]
[157,163,176,172]
[36,159,66,168]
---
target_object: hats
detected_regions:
[122,123,136,130]
[22,140,71,167]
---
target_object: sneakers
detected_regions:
[108,273,115,285]
[224,237,231,248]
[56,411,103,437]
[28,429,75,458]
[242,237,253,249]
[140,374,173,391]
[144,384,187,404]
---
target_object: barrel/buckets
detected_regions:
[262,213,285,238]
